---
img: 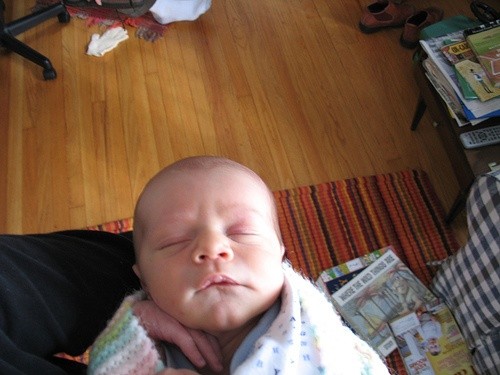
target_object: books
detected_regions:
[314,244,478,375]
[419,19,500,128]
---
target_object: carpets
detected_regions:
[57,171,461,369]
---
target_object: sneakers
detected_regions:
[359,0,416,34]
[400,7,444,49]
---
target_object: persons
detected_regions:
[0,228,145,375]
[86,155,393,375]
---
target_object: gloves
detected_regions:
[86,26,130,56]
